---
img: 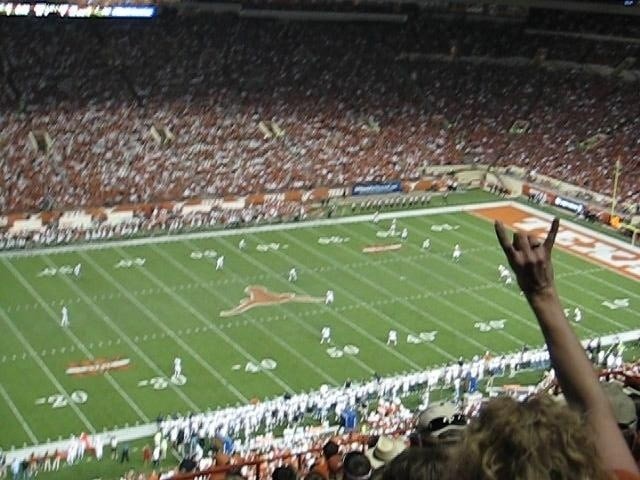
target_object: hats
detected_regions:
[602,380,637,435]
[418,401,469,437]
[365,434,406,469]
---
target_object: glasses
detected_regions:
[426,413,467,434]
